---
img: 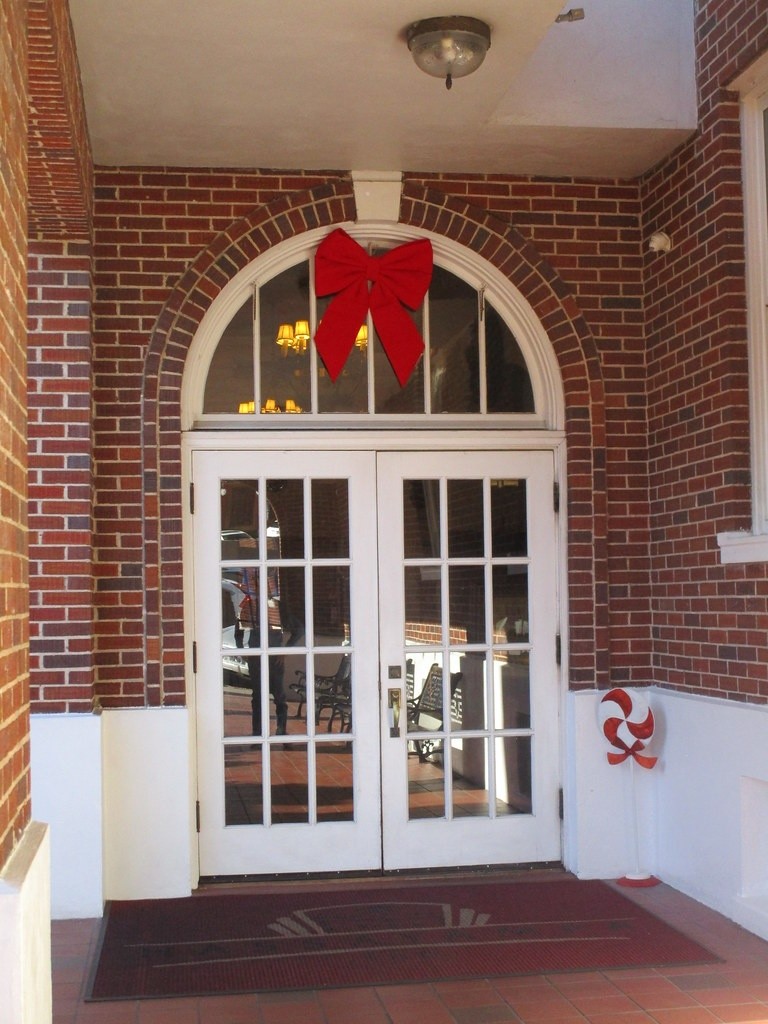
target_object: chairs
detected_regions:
[290,655,463,763]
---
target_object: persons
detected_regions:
[235,574,304,749]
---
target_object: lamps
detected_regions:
[237,399,304,414]
[407,15,492,92]
[276,319,369,357]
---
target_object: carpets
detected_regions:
[82,878,728,1004]
